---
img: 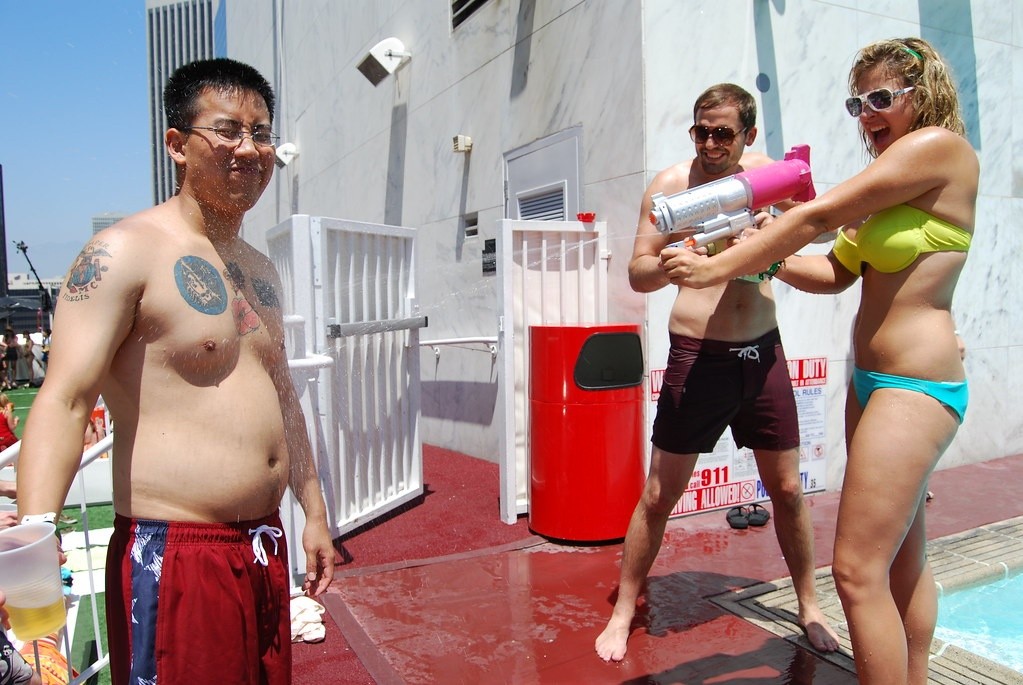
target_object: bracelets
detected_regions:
[954,330,960,335]
[759,261,782,281]
[21,512,62,546]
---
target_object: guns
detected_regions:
[664,204,760,258]
[650,143,816,237]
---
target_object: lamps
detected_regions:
[274,142,299,169]
[356,37,413,88]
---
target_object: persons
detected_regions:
[594,83,839,662]
[36,307,41,331]
[0,392,105,685]
[41,328,52,372]
[21,329,35,388]
[0,320,21,391]
[18,59,338,685]
[657,36,979,685]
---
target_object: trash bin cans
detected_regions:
[528,325,645,542]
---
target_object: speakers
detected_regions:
[273,143,296,169]
[355,37,405,88]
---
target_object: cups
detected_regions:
[0,522,66,642]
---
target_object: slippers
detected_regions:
[748,503,770,526]
[726,507,749,528]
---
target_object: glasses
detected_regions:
[181,126,280,147]
[845,87,915,118]
[688,124,747,146]
